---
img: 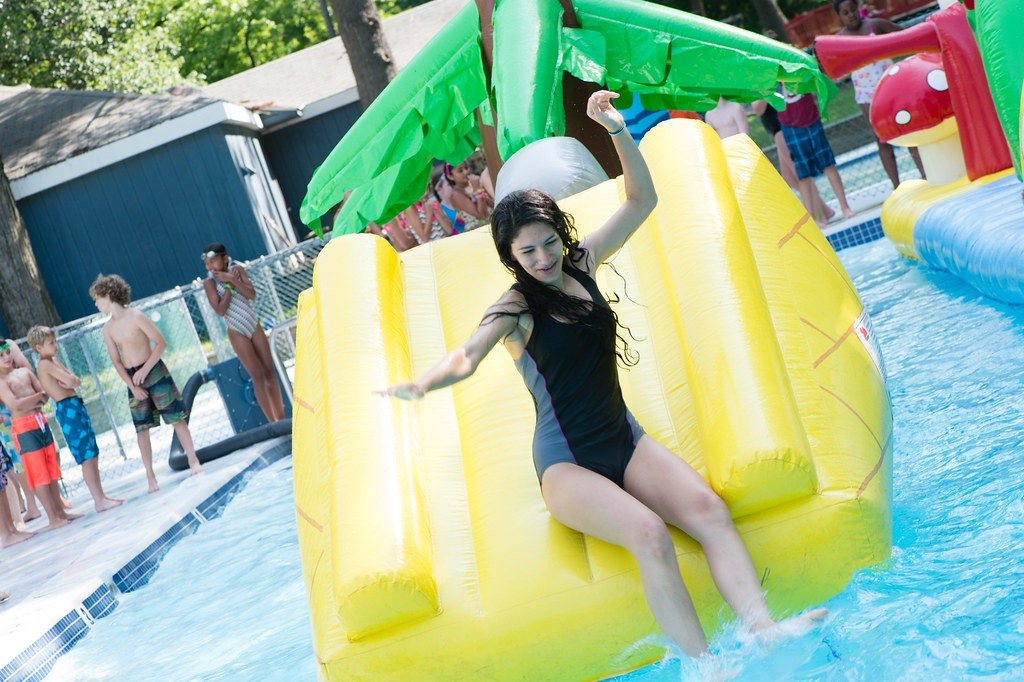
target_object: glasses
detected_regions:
[0,342,10,351]
[199,251,222,259]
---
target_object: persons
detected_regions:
[705,95,748,141]
[773,82,856,230]
[0,339,85,528]
[89,272,203,495]
[0,439,27,514]
[859,0,888,20]
[834,0,927,190]
[202,243,287,424]
[27,325,124,514]
[750,98,836,227]
[372,89,829,682]
[334,155,496,254]
[0,335,72,523]
[0,473,38,551]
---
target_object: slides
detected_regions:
[291,118,894,682]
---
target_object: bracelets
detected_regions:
[608,123,626,137]
